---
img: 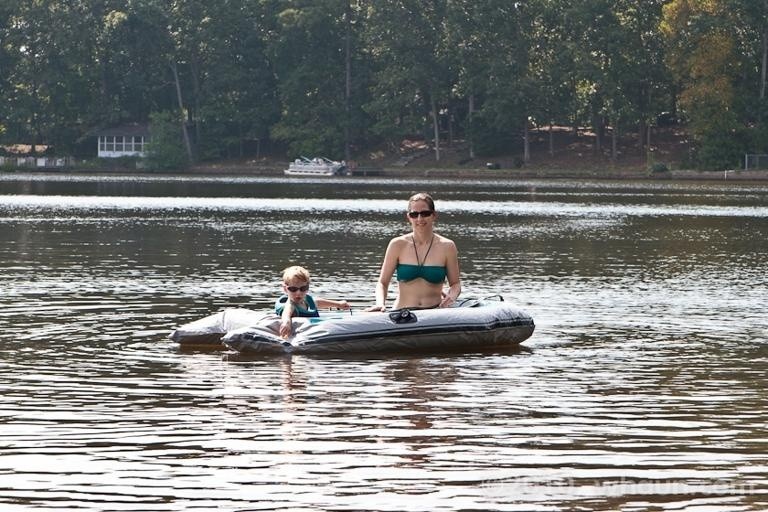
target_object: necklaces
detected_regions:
[410,232,434,278]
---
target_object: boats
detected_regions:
[169,297,536,361]
[284,155,346,177]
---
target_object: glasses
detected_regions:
[408,210,434,220]
[286,285,310,293]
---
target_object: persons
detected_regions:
[361,192,461,311]
[274,265,352,338]
